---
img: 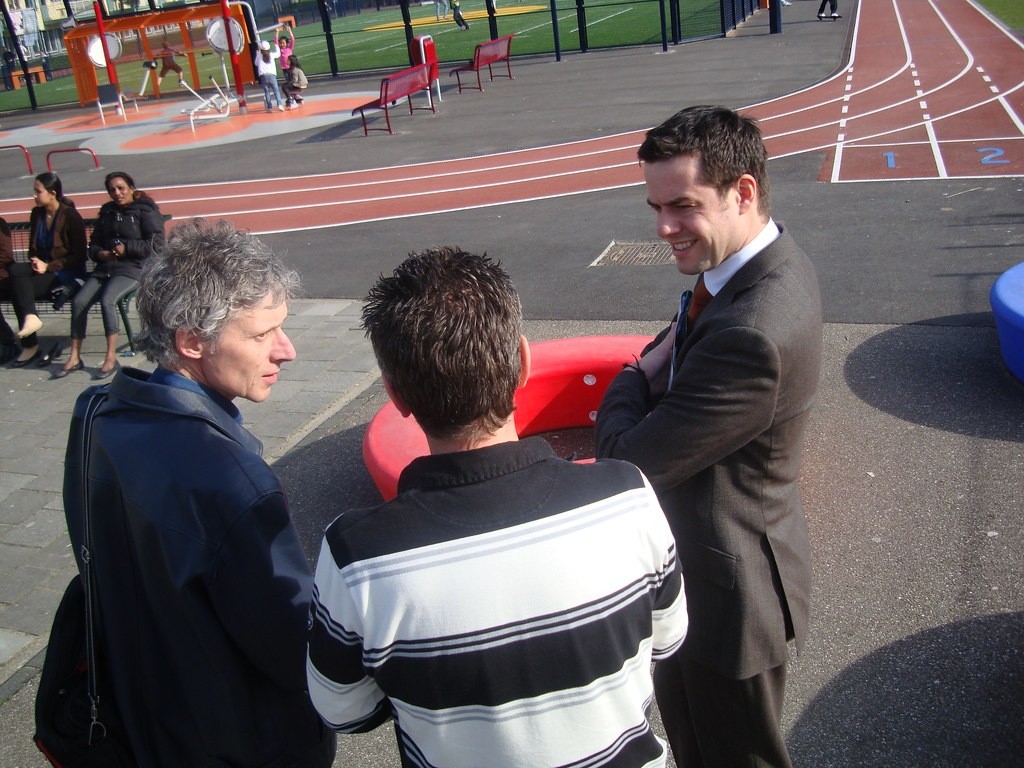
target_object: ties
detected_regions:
[687,279,710,318]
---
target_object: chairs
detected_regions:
[96,83,126,126]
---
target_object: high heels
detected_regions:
[12,349,41,366]
[38,343,62,365]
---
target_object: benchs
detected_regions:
[352,58,440,136]
[449,34,517,94]
[0,214,171,356]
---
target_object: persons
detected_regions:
[434,0,449,21]
[33,218,338,768]
[592,104,823,768]
[254,40,285,113]
[7,173,89,367]
[818,0,838,17]
[448,0,470,31]
[53,172,166,380]
[282,55,308,110]
[274,26,304,103]
[306,247,690,768]
[153,41,188,88]
[0,216,21,366]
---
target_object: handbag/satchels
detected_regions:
[37,568,111,768]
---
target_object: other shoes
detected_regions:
[294,93,304,102]
[461,25,469,32]
[443,16,447,19]
[818,13,825,17]
[178,82,187,86]
[288,102,298,109]
[52,358,83,376]
[437,18,439,21]
[96,360,122,379]
[831,13,838,16]
[279,104,285,111]
[265,107,273,113]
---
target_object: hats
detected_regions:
[260,39,270,49]
